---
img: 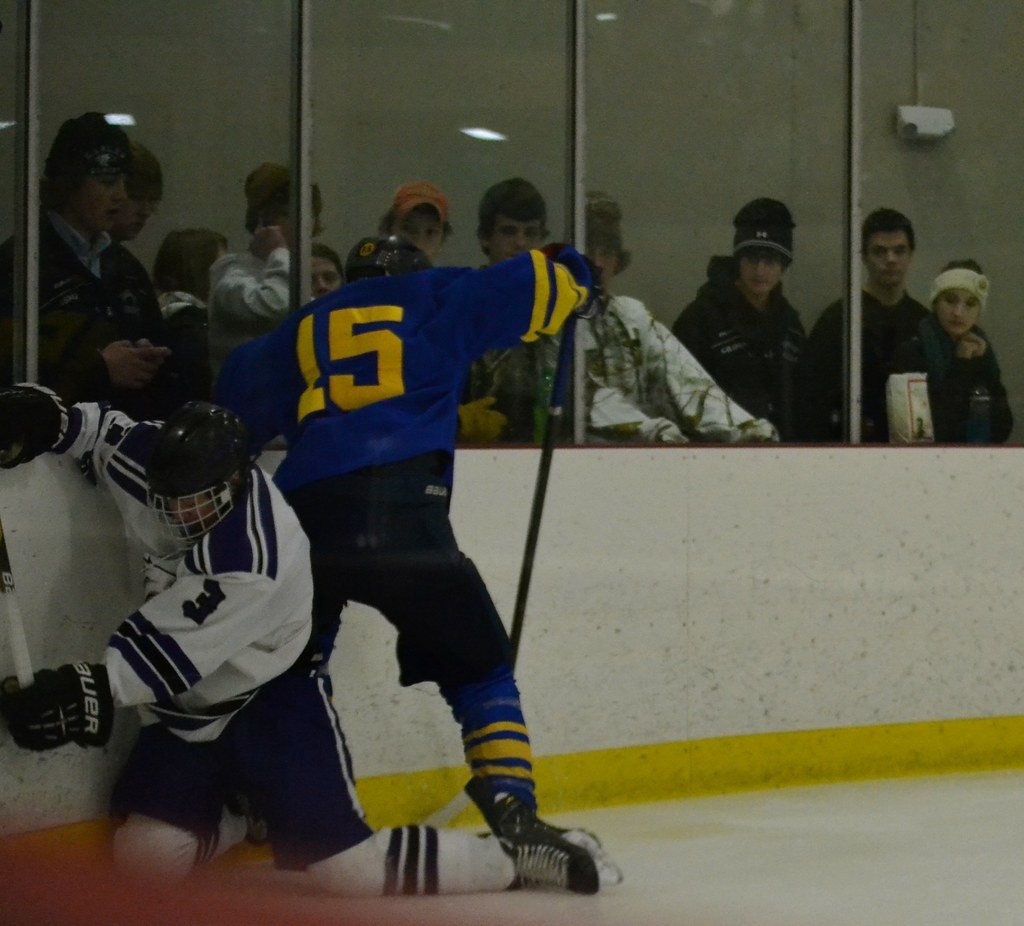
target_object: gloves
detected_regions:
[457,397,507,443]
[0,382,82,469]
[576,253,611,321]
[0,661,115,752]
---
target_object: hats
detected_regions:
[392,181,449,226]
[732,198,796,263]
[586,190,630,274]
[930,258,988,314]
[244,162,322,235]
[45,113,138,174]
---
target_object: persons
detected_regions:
[309,241,342,300]
[226,237,610,836]
[672,197,808,442]
[549,191,778,445]
[913,260,1014,446]
[470,178,548,438]
[804,207,930,441]
[152,228,227,397]
[1,381,620,894]
[1,112,173,418]
[380,179,506,441]
[208,165,324,371]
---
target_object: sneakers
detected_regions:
[477,829,625,896]
[464,774,602,847]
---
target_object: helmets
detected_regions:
[144,400,249,551]
[343,236,431,282]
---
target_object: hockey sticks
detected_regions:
[421,309,578,827]
[0,516,35,690]
